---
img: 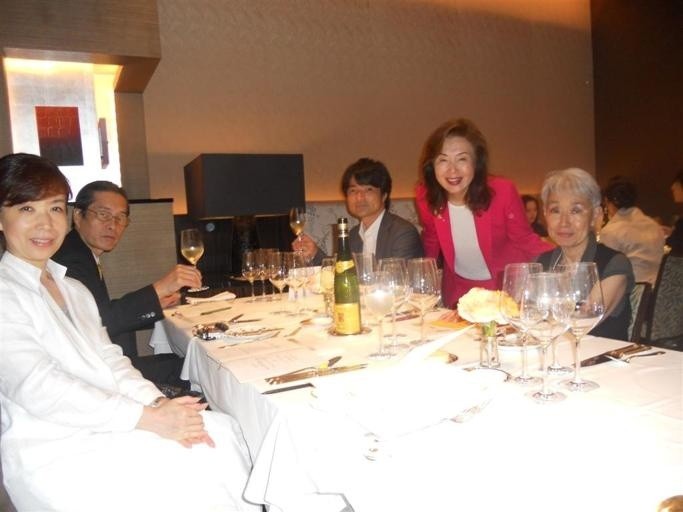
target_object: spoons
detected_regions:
[265,355,343,380]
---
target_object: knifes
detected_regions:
[571,343,641,368]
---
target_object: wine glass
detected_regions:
[500,260,606,402]
[180,227,210,293]
[320,257,336,319]
[354,252,441,360]
[242,248,315,320]
[290,207,307,253]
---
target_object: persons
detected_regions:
[413,116,557,310]
[663,174,683,255]
[594,170,664,288]
[48,180,202,397]
[0,151,266,512]
[290,157,422,284]
[529,168,634,339]
[516,195,550,240]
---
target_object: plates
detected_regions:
[225,322,280,341]
[476,340,541,350]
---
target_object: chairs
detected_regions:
[628,254,682,352]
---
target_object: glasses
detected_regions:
[89,210,132,226]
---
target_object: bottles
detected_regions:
[332,216,362,337]
